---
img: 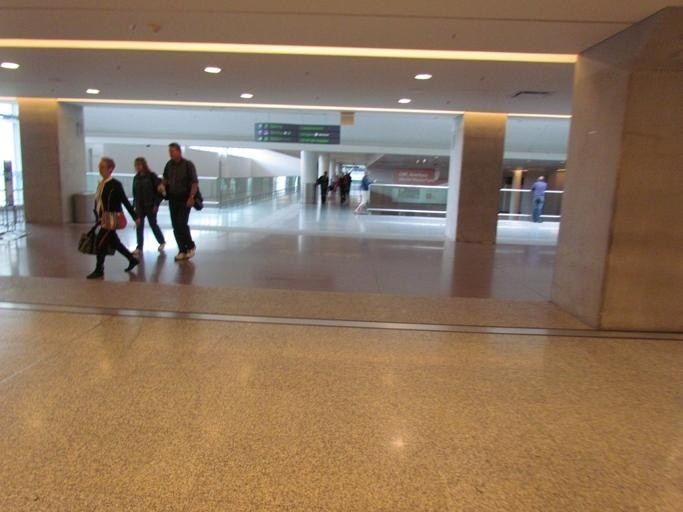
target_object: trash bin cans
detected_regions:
[73,193,96,224]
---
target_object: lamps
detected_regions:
[339,111,353,124]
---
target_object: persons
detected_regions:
[130,158,166,255]
[86,157,141,278]
[163,143,203,260]
[529,175,548,223]
[315,170,352,204]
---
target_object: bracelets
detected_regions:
[190,195,194,199]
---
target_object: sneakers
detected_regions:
[86,267,105,279]
[132,245,144,254]
[157,241,167,251]
[124,258,139,272]
[186,244,197,259]
[175,252,186,260]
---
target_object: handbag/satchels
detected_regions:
[191,184,204,210]
[100,210,126,229]
[78,225,116,256]
